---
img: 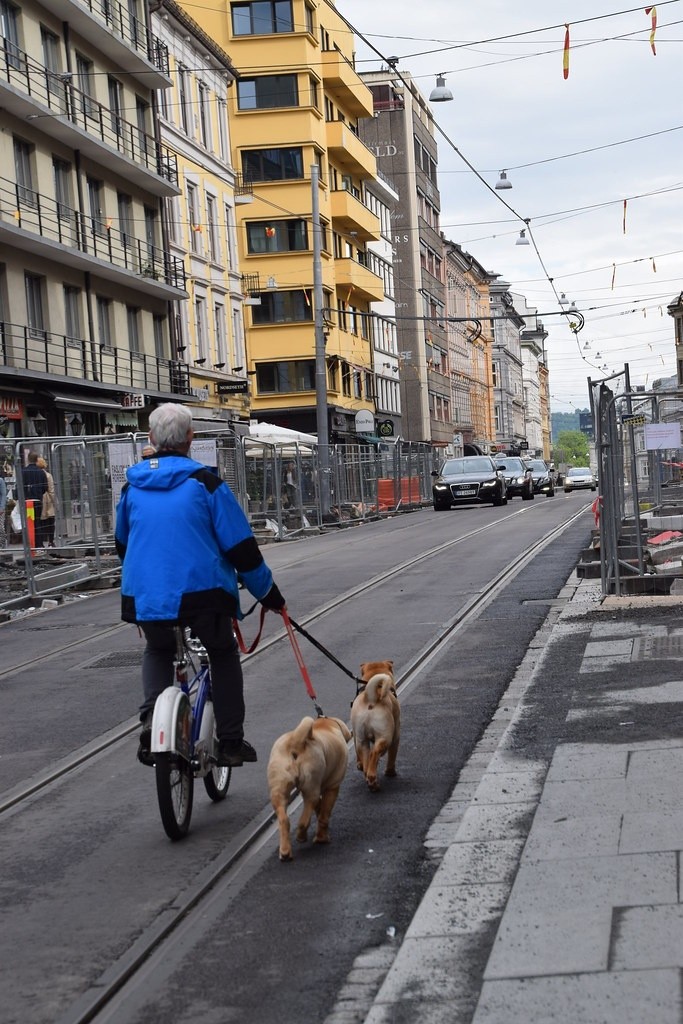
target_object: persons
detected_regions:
[0,450,59,552]
[113,403,290,767]
[284,461,299,507]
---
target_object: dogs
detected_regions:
[351,660,401,789]
[266,715,352,861]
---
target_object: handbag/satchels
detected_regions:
[10,500,22,533]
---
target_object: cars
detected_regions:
[429,456,510,512]
[561,466,597,493]
[523,459,556,497]
[492,457,534,500]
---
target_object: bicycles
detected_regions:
[149,623,233,841]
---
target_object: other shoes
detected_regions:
[36,546,45,549]
[48,542,56,547]
[217,739,257,767]
[137,708,154,767]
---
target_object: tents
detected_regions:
[240,421,319,457]
